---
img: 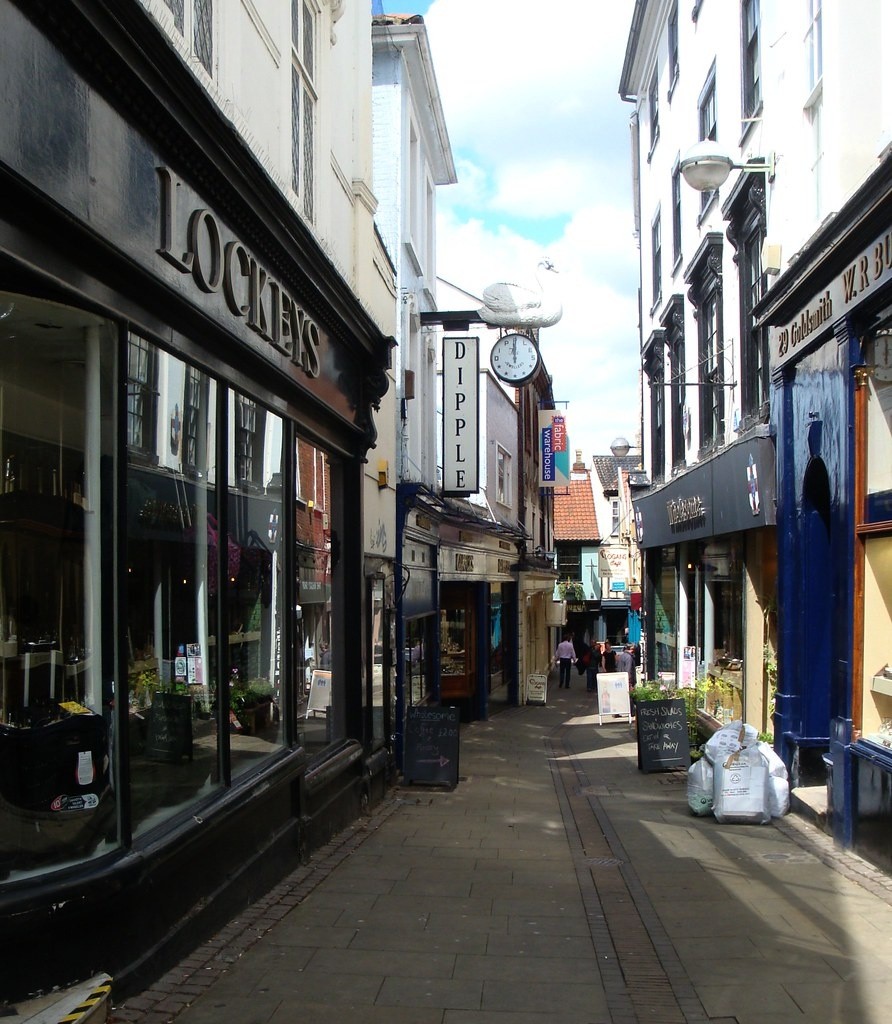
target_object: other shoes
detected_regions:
[559,685,562,688]
[565,687,570,689]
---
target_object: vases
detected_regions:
[567,586,575,595]
[243,702,270,735]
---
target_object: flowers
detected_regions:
[559,576,584,601]
[230,668,279,727]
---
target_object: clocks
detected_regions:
[490,334,540,383]
[871,332,892,383]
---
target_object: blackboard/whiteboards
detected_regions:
[635,698,692,773]
[403,705,461,788]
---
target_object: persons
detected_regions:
[412,637,424,676]
[555,634,577,688]
[586,640,637,718]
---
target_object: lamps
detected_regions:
[610,438,642,457]
[679,139,778,193]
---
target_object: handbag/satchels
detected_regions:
[582,652,590,667]
